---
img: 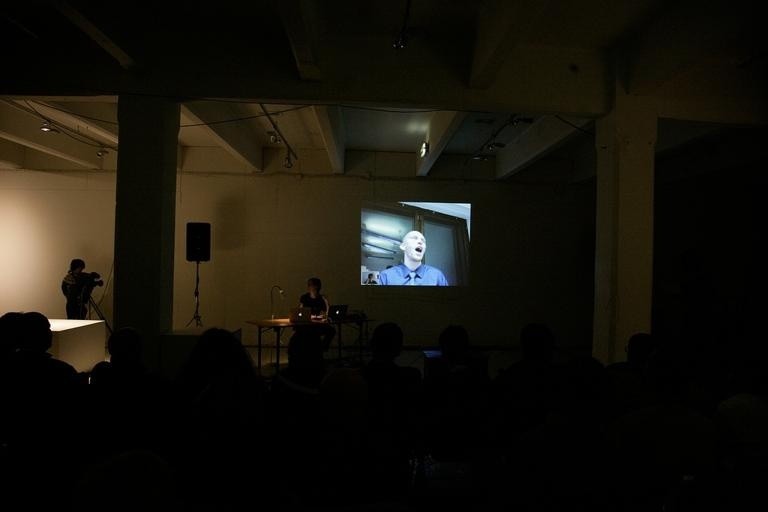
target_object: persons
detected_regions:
[378,230,448,286]
[62,259,87,320]
[364,273,377,285]
[0,312,768,423]
[299,277,336,352]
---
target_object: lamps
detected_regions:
[269,128,296,169]
[270,284,286,318]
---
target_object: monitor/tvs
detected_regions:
[407,344,443,359]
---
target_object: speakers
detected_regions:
[185,223,211,262]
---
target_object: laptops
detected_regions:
[322,304,348,321]
[288,307,312,323]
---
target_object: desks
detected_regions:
[248,318,377,374]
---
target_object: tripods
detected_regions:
[185,261,205,328]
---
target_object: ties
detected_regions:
[408,271,417,286]
